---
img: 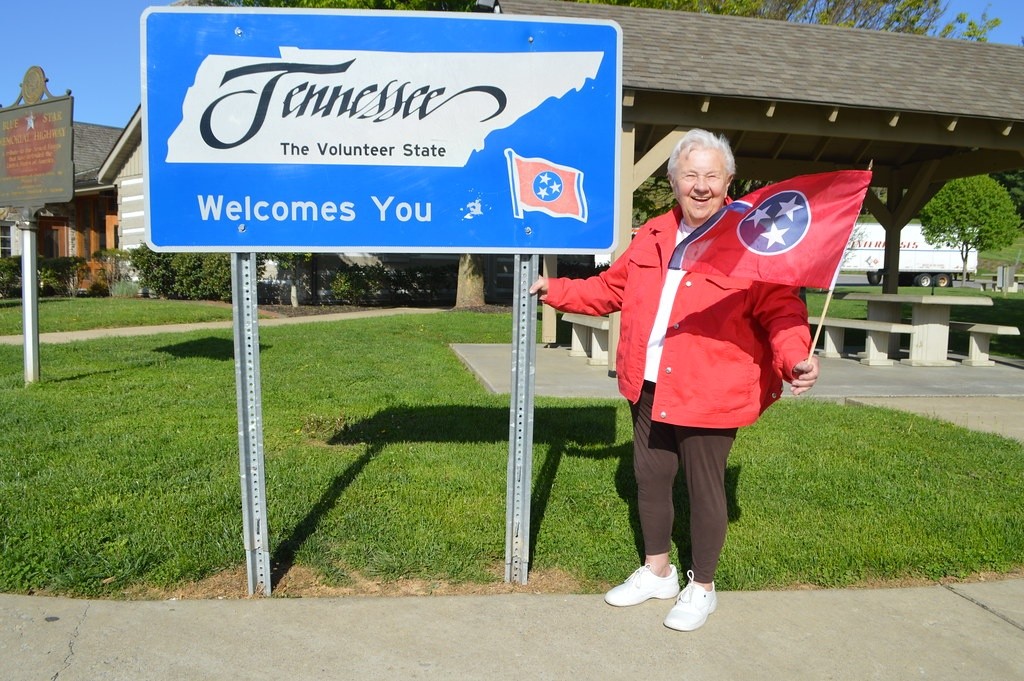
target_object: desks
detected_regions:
[982,274,1024,293]
[843,293,993,366]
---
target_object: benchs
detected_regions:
[561,312,609,367]
[809,316,917,366]
[904,317,1020,368]
[976,280,997,292]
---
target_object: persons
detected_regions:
[531,129,819,633]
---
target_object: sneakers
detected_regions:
[604,564,680,607]
[664,570,717,631]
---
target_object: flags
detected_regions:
[667,170,873,289]
